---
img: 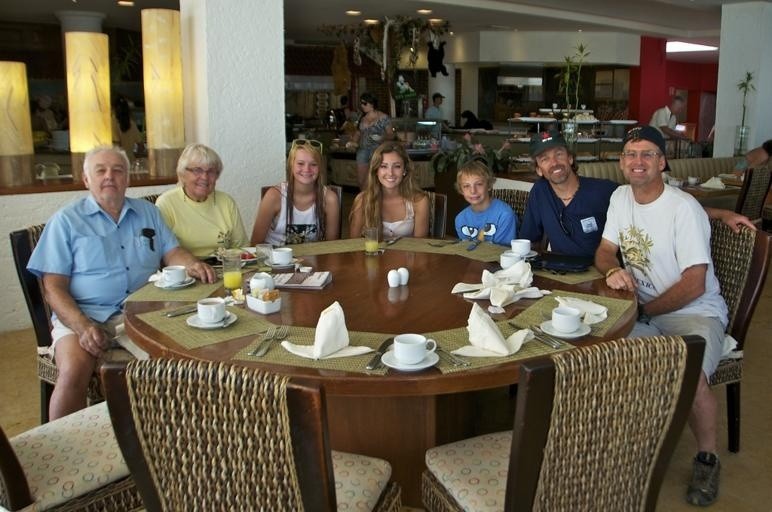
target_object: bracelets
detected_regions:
[606,267,623,279]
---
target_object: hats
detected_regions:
[432,92,445,100]
[618,125,672,176]
[528,128,569,158]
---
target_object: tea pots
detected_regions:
[34,160,62,185]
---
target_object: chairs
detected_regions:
[1,397,149,511]
[101,353,401,511]
[421,334,707,511]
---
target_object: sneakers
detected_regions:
[683,449,722,507]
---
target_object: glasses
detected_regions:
[292,137,323,153]
[614,149,661,163]
[186,167,220,179]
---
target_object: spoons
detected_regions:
[223,310,230,328]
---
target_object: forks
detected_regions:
[528,322,560,346]
[248,324,290,358]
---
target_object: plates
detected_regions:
[540,320,592,338]
[380,348,442,372]
[187,312,237,329]
[155,276,197,289]
[227,247,264,262]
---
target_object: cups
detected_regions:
[503,249,538,257]
[163,265,186,283]
[272,247,293,266]
[551,306,583,333]
[197,297,225,321]
[364,228,379,256]
[499,253,522,269]
[133,159,145,172]
[670,176,703,189]
[250,273,274,293]
[256,243,272,272]
[395,333,438,363]
[511,239,531,255]
[223,253,242,290]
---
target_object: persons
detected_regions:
[518,130,761,263]
[251,140,342,249]
[595,126,731,508]
[425,93,446,138]
[31,90,142,164]
[24,144,219,423]
[155,144,251,261]
[397,75,416,94]
[345,93,394,192]
[649,97,687,141]
[322,95,356,131]
[734,139,771,179]
[460,110,495,129]
[349,141,433,239]
[454,160,521,249]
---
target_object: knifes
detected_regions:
[366,336,394,369]
[508,322,558,350]
